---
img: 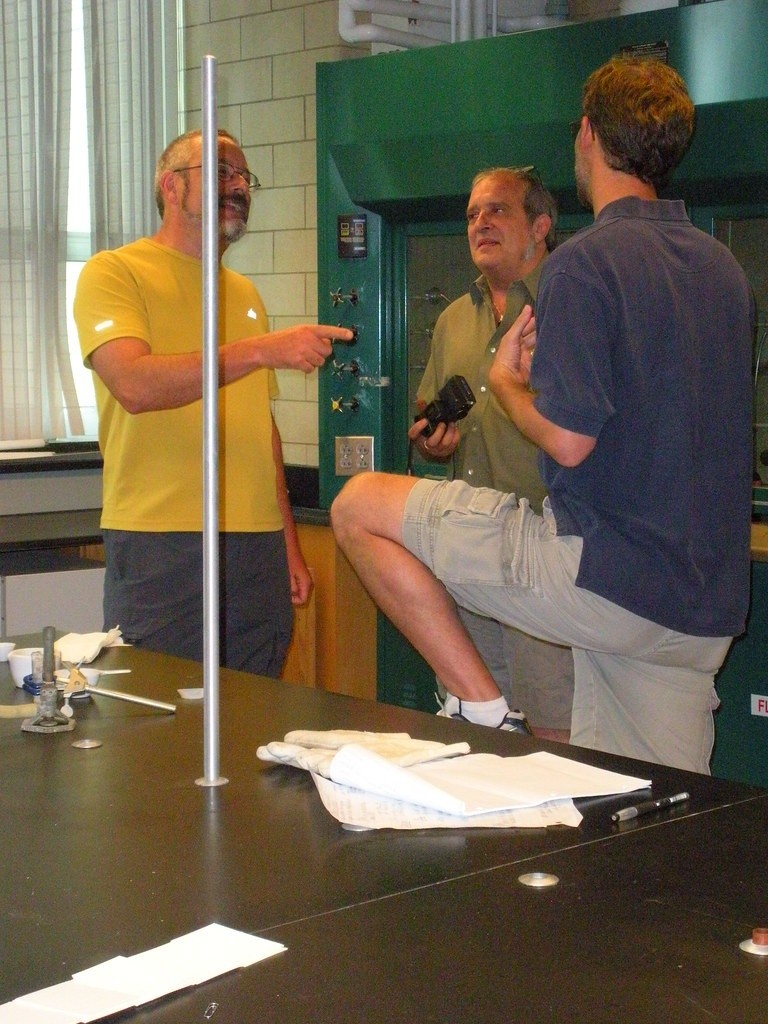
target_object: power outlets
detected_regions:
[335,436,374,476]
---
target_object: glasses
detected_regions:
[569,115,596,142]
[509,164,553,218]
[172,162,261,192]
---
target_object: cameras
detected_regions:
[414,374,476,437]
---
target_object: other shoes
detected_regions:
[430,691,534,738]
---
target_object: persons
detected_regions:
[328,52,752,780]
[406,166,574,743]
[73,126,355,680]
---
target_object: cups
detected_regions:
[0,642,16,662]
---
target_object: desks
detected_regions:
[0,628,768,1024]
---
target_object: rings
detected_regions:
[424,440,432,450]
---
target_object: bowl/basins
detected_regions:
[7,648,61,690]
[55,668,101,698]
